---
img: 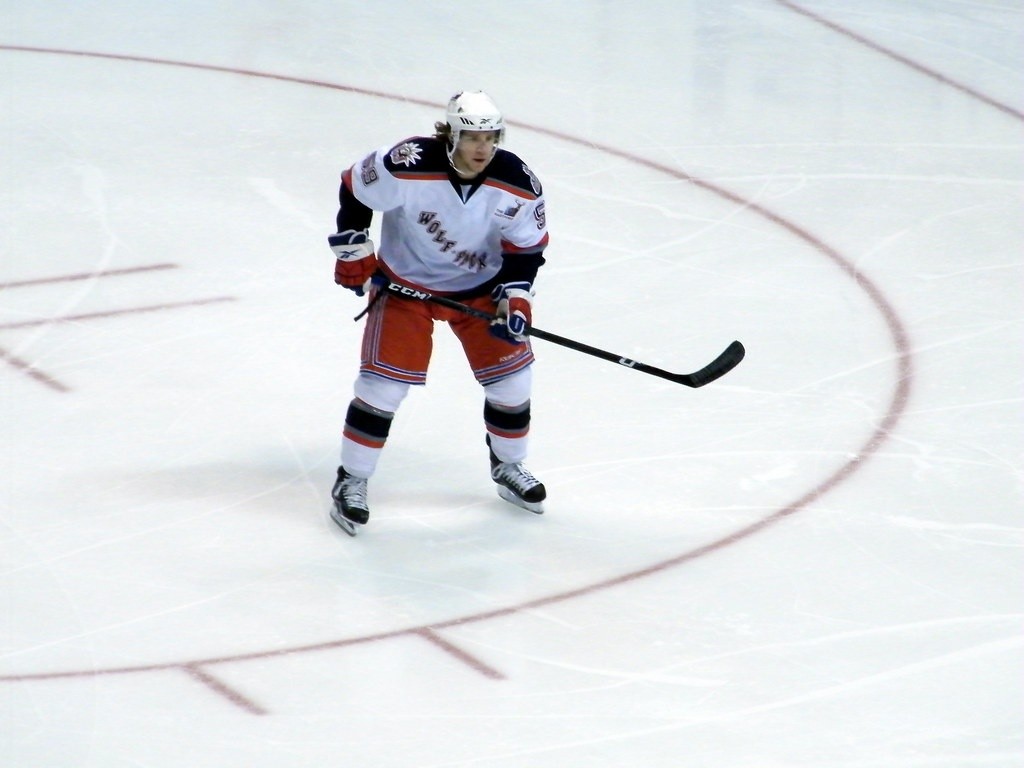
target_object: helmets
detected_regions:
[447,91,505,148]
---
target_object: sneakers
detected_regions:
[330,466,370,536]
[486,433,546,514]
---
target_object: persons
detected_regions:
[326,88,551,538]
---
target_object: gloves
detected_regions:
[490,282,533,345]
[328,229,380,297]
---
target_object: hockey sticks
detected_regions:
[371,272,747,390]
